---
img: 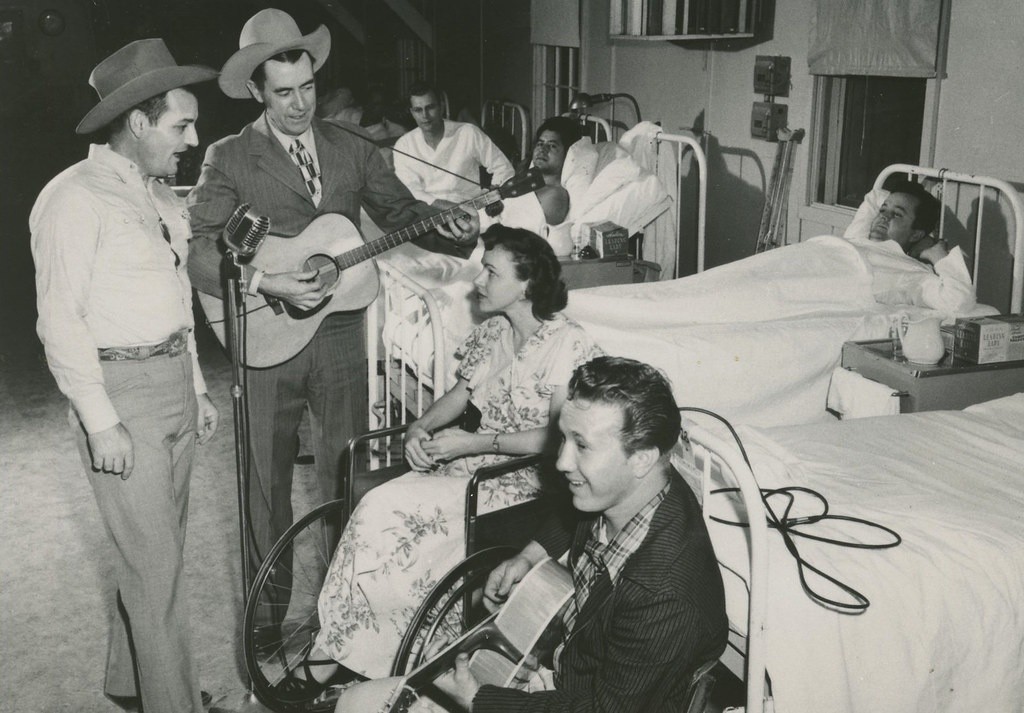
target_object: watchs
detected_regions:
[493,434,499,451]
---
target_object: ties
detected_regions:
[289,139,323,199]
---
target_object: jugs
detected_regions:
[897,310,947,365]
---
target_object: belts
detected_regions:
[96,332,188,362]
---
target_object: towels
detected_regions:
[826,365,901,420]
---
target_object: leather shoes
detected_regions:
[252,625,283,664]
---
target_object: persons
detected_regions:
[29,39,222,713]
[333,357,729,713]
[274,223,604,703]
[842,181,976,315]
[518,116,581,224]
[391,82,515,207]
[333,85,393,169]
[184,8,479,663]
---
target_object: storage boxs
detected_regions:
[955,314,1024,364]
[582,222,628,260]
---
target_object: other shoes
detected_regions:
[107,689,211,710]
[272,646,357,704]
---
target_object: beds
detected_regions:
[177,99,1024,713]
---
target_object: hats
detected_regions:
[75,39,222,135]
[219,8,332,99]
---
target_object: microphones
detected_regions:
[223,201,271,302]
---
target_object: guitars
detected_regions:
[197,165,546,370]
[377,556,577,713]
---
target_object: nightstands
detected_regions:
[556,254,633,290]
[842,334,1024,412]
